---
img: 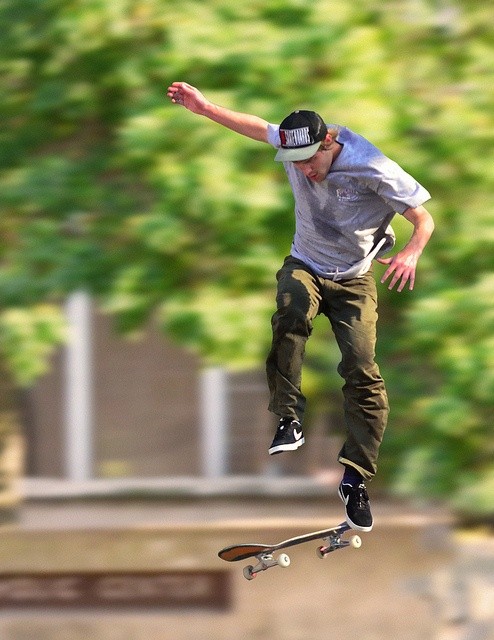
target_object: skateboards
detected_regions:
[218,521,362,580]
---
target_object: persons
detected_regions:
[166,80,434,533]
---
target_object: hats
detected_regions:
[273,110,328,162]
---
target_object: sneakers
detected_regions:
[338,480,373,534]
[268,416,305,455]
